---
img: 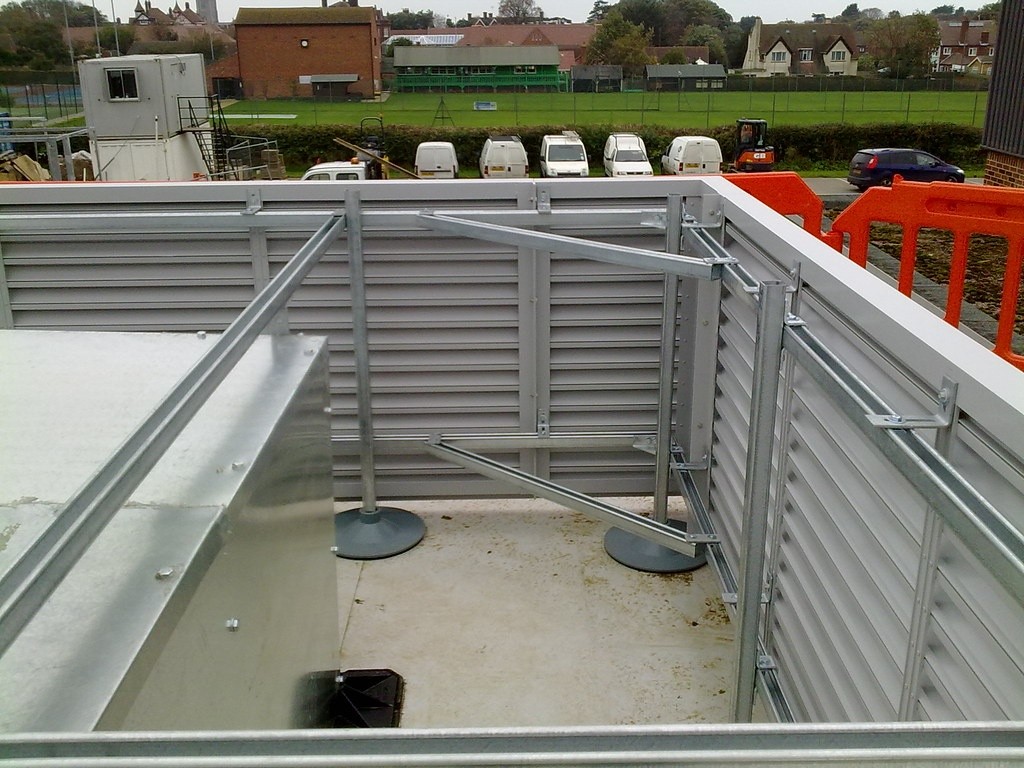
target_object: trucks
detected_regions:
[479,133,529,178]
[603,132,654,177]
[539,131,591,176]
[660,136,723,175]
[414,141,459,178]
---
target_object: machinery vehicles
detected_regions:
[354,117,390,180]
[728,117,774,173]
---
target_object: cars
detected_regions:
[299,157,383,180]
[847,147,965,190]
